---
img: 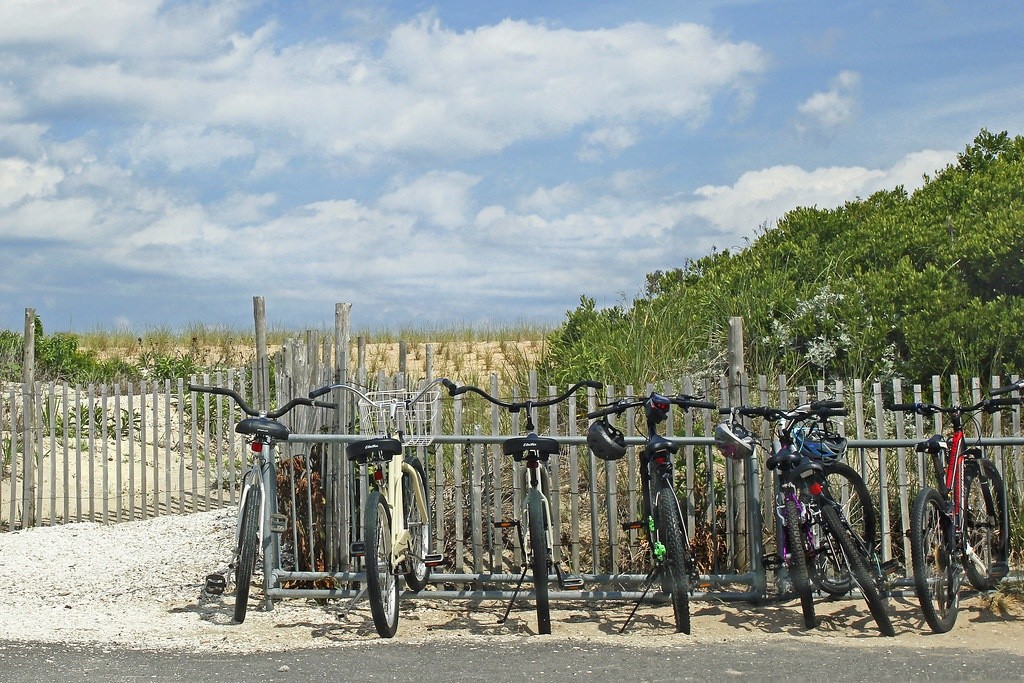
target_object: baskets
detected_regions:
[358,388,440,447]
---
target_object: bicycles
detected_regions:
[889,378,1024,635]
[309,376,457,638]
[188,383,339,623]
[586,393,718,636]
[450,380,603,636]
[722,399,897,640]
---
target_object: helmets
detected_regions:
[588,419,626,460]
[715,418,756,461]
[792,426,847,465]
